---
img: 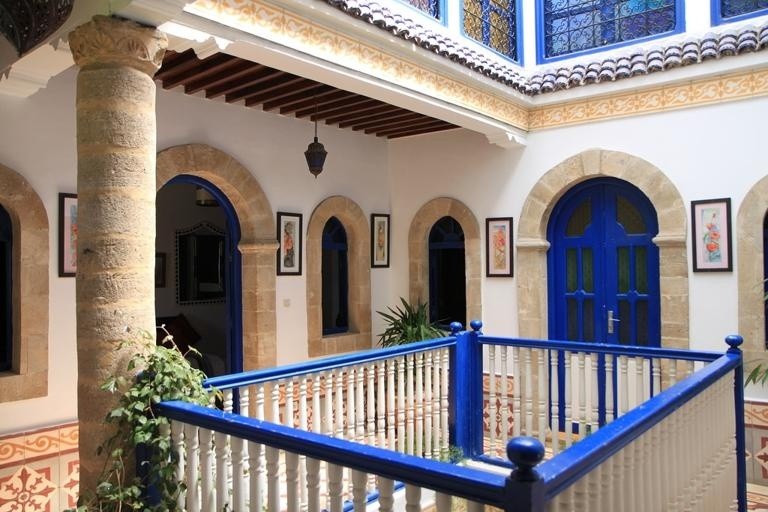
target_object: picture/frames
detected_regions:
[691,197,733,275]
[486,218,513,277]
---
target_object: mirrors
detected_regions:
[370,213,390,269]
[174,220,224,306]
[59,193,77,277]
[277,212,304,277]
[156,252,166,288]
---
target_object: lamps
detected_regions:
[304,97,328,179]
